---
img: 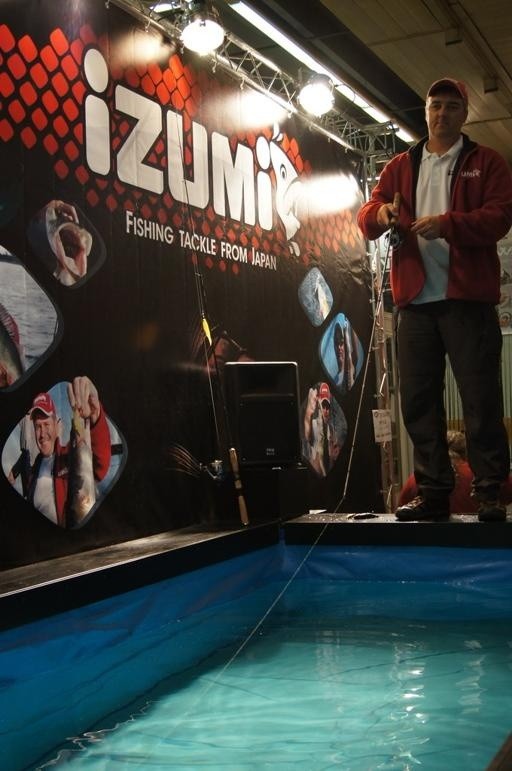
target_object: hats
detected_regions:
[29,394,53,416]
[334,313,345,338]
[318,382,331,404]
[427,78,467,98]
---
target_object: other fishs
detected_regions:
[318,413,330,477]
[0,302,25,388]
[342,322,356,391]
[64,409,99,529]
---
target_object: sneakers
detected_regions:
[478,500,506,521]
[396,496,451,521]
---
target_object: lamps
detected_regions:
[180,15,336,117]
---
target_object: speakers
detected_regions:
[223,361,303,466]
[218,466,310,522]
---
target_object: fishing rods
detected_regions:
[344,193,399,499]
[183,180,252,528]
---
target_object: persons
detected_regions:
[329,314,358,390]
[7,377,111,526]
[358,78,512,521]
[304,383,339,475]
